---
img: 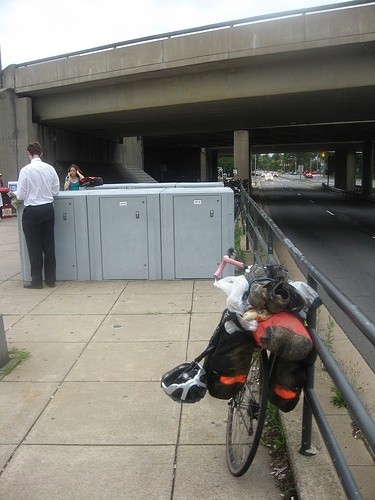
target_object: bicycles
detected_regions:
[210,248,269,478]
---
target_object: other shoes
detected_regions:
[247,266,305,312]
[24,282,43,289]
[45,281,55,287]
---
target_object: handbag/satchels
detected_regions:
[80,176,101,186]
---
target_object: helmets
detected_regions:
[160,362,207,404]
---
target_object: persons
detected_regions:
[7,142,59,289]
[64,164,85,191]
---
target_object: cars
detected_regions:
[252,169,278,181]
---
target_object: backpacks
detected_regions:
[204,309,256,400]
[269,348,306,413]
